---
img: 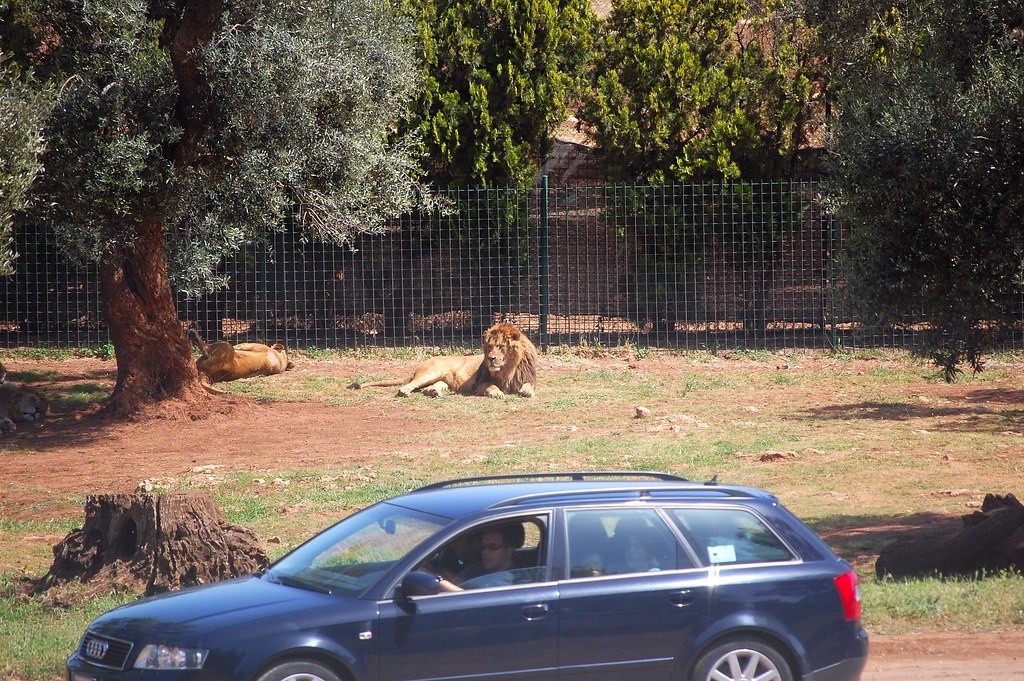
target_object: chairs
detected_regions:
[567,514,674,576]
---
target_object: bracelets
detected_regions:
[435,575,448,583]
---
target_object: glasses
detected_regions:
[480,543,507,551]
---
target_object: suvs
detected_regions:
[64,469,871,681]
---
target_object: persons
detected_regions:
[416,525,530,592]
[568,516,661,577]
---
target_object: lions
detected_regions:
[347,323,537,400]
[188,329,296,396]
[0,383,48,436]
